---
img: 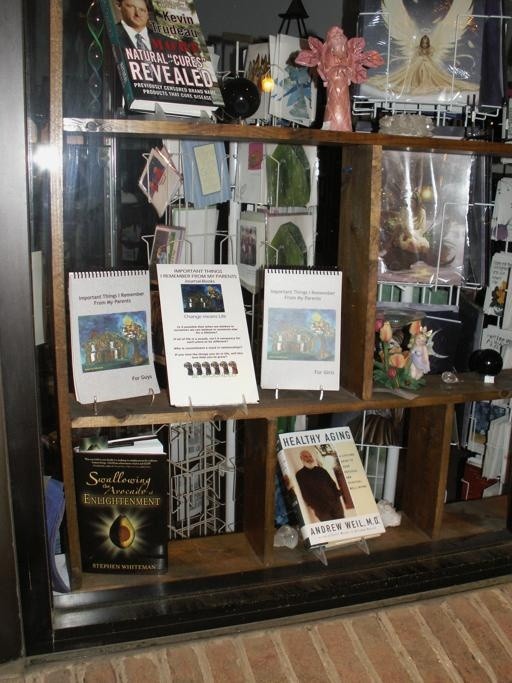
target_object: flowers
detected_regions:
[372,318,430,392]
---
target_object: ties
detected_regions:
[135,33,149,52]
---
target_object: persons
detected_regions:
[378,185,430,258]
[295,449,347,523]
[406,30,440,94]
[117,0,212,103]
[405,321,447,382]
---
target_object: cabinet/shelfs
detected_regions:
[43,0,512,594]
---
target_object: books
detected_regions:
[260,264,342,392]
[156,264,260,408]
[75,435,170,576]
[276,424,387,552]
[100,0,226,117]
[69,270,161,404]
[378,148,487,290]
[354,0,503,108]
